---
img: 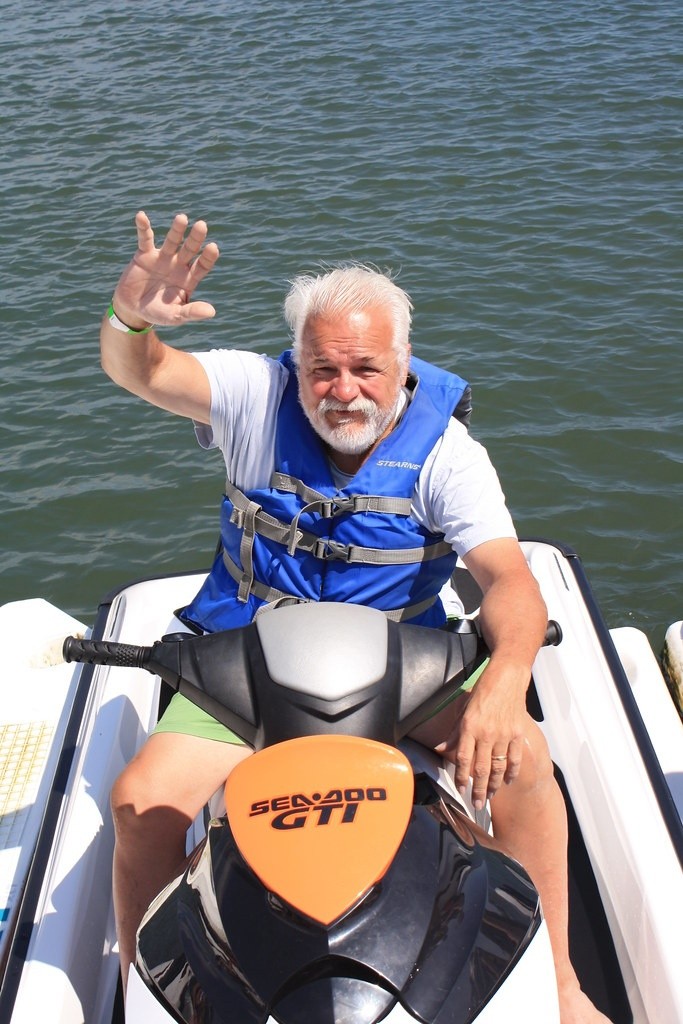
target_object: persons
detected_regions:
[101,210,613,1024]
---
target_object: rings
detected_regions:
[492,756,507,760]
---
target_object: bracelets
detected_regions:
[107,298,155,335]
